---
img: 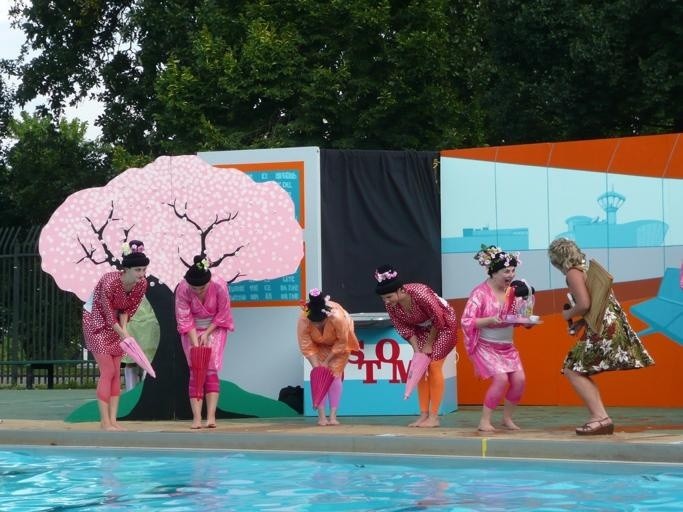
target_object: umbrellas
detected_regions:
[191,336,212,402]
[119,336,156,378]
[404,352,432,400]
[310,366,335,410]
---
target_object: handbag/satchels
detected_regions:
[584,259,613,334]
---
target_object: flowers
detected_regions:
[299,285,333,318]
[474,242,520,271]
[120,240,146,256]
[198,255,213,273]
[374,269,398,283]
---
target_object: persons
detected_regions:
[376,265,458,427]
[297,288,355,426]
[461,244,535,431]
[548,237,655,435]
[82,240,149,431]
[175,255,230,429]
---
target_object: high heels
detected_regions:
[575,417,613,435]
